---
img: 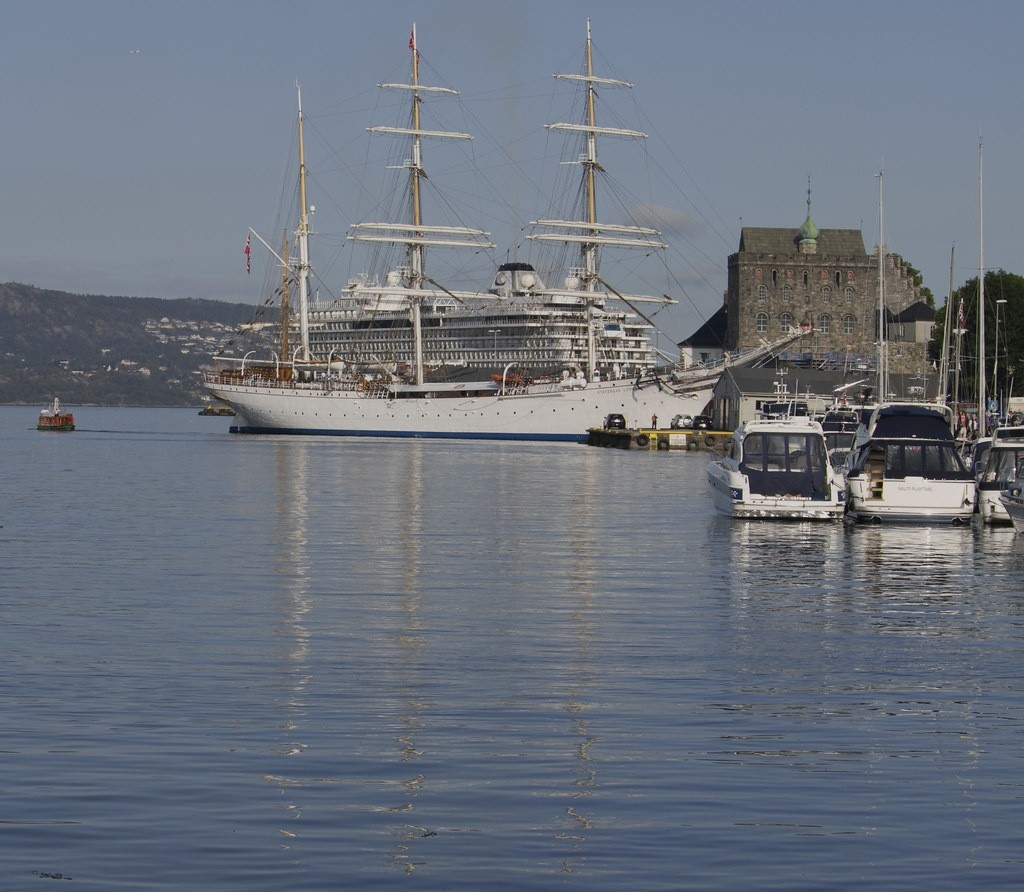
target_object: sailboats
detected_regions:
[201,16,812,442]
[705,134,1024,535]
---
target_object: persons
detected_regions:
[652,414,658,429]
[958,410,1014,438]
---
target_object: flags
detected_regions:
[958,303,965,329]
[409,29,419,77]
[244,234,251,275]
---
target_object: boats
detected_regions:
[36,397,76,431]
[198,403,237,416]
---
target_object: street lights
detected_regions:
[991,299,1007,426]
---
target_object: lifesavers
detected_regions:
[724,438,731,449]
[688,440,698,450]
[637,434,648,446]
[658,439,669,449]
[705,435,716,446]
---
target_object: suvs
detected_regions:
[671,414,693,429]
[603,413,626,430]
[694,415,712,430]
